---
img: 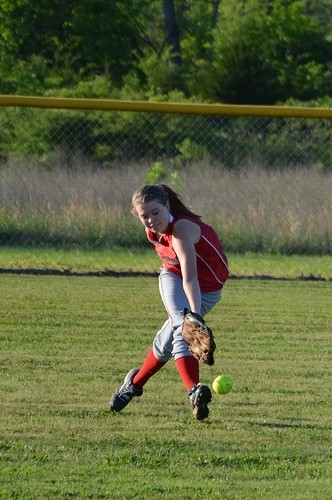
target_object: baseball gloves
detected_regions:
[181,307,215,366]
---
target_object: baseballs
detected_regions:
[214,375,232,393]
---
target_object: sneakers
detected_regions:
[108,368,144,412]
[188,383,212,420]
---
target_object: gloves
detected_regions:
[182,308,216,366]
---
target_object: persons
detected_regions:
[109,184,230,420]
